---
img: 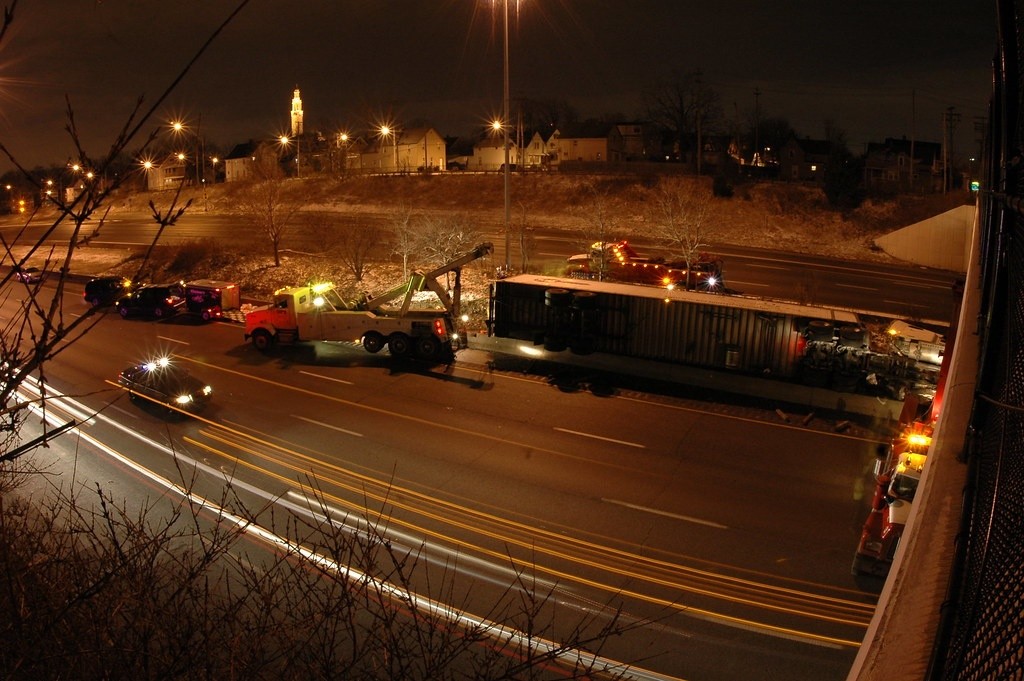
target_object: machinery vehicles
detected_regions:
[243,239,497,373]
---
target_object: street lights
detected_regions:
[280,136,301,179]
[172,122,205,185]
[382,124,396,173]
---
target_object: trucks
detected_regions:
[565,238,726,293]
[851,384,938,587]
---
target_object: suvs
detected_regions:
[116,280,185,322]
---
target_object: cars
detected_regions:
[119,359,214,417]
[16,266,45,285]
[84,272,145,307]
